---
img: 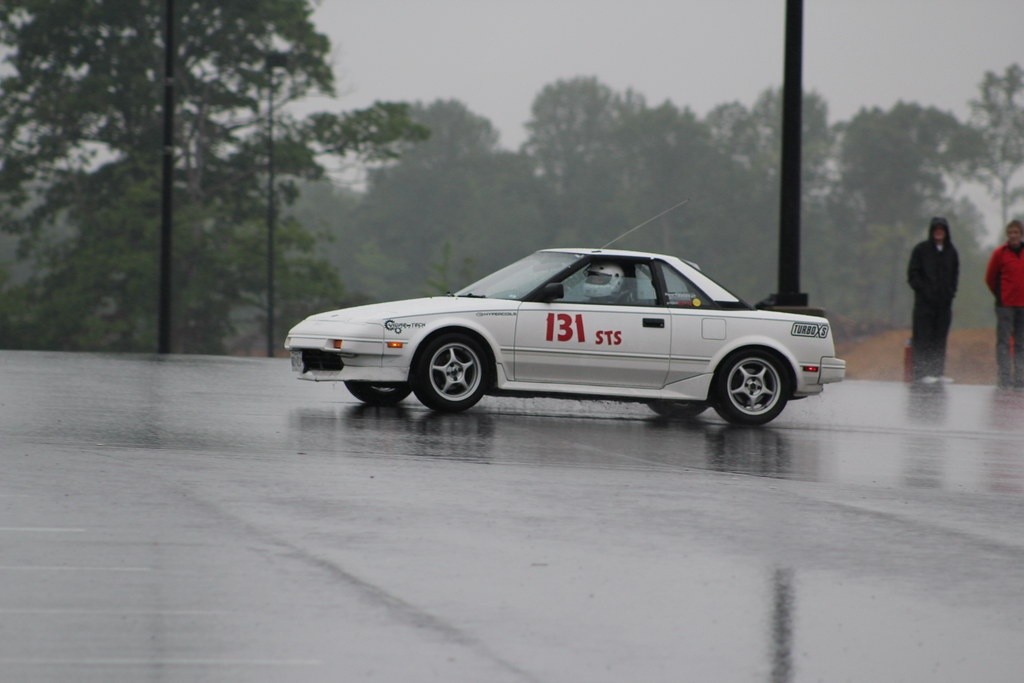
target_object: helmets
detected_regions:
[583,262,624,297]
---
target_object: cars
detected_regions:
[283,248,847,428]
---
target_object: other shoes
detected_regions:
[997,382,1013,393]
[914,375,937,384]
[936,376,954,383]
[1014,380,1024,392]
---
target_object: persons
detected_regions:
[907,217,960,383]
[986,220,1024,389]
[583,263,625,305]
